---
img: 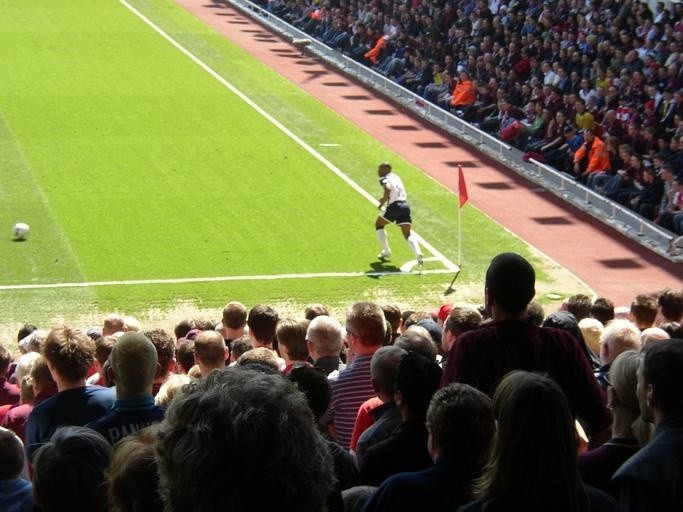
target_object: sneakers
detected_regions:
[417,254,423,264]
[377,251,392,258]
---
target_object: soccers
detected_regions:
[13,222,30,240]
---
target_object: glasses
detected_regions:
[603,372,613,387]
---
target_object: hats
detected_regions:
[416,319,441,343]
[432,304,453,320]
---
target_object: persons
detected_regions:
[374,161,423,265]
[438,253,613,452]
[260,0,682,234]
[0,293,682,511]
